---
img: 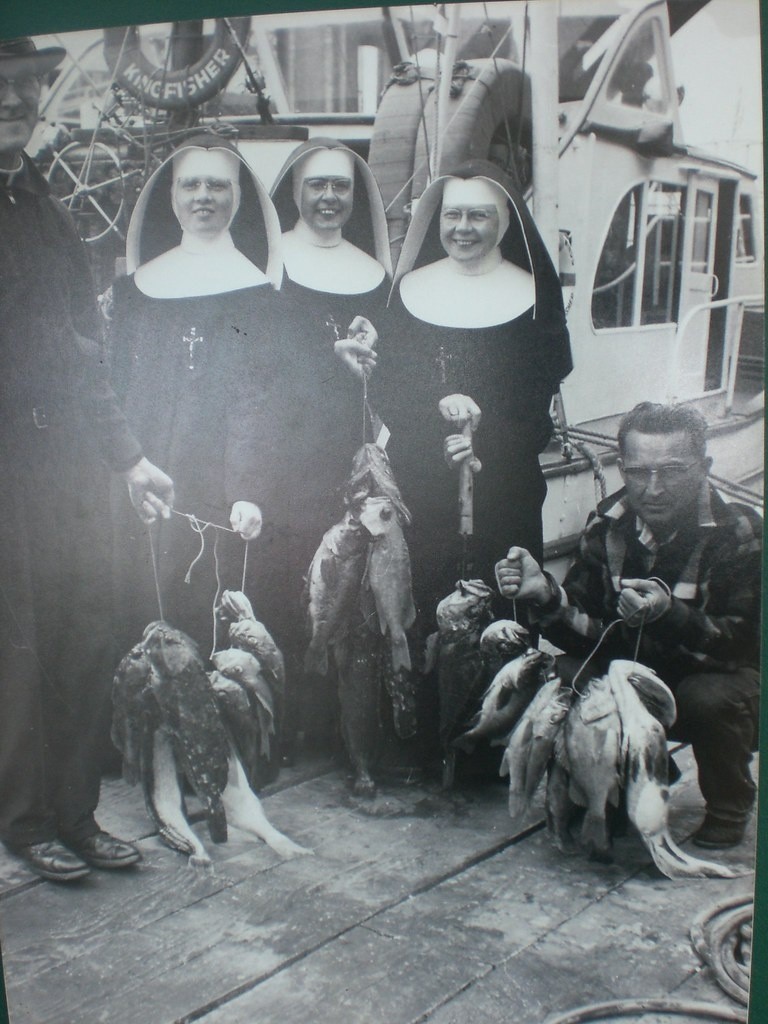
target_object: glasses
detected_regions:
[621,459,701,482]
[295,177,353,194]
[175,176,230,193]
[0,72,44,101]
[442,207,496,226]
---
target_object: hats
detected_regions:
[0,37,67,72]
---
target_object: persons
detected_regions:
[0,37,575,882]
[493,401,764,850]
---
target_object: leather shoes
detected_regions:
[18,838,91,882]
[69,831,143,869]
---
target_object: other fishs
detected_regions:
[305,443,420,797]
[420,580,753,879]
[106,589,314,868]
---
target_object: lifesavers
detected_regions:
[101,13,253,113]
[363,42,538,285]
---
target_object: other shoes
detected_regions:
[694,816,746,847]
